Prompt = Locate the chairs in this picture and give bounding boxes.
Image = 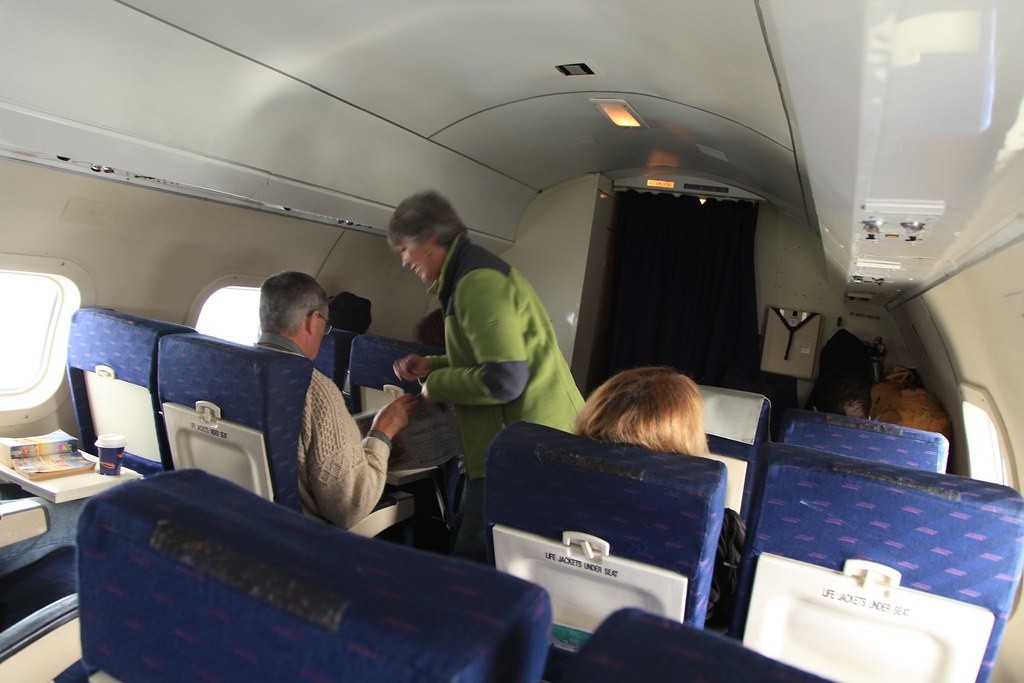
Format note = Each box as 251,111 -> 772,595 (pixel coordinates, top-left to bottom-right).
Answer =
1,305 -> 1024,683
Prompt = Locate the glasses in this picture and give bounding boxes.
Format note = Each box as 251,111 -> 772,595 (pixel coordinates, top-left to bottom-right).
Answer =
308,312 -> 334,335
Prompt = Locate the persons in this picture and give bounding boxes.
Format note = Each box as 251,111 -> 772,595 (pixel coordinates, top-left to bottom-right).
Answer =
253,272 -> 422,543
569,366 -> 746,633
385,188 -> 586,563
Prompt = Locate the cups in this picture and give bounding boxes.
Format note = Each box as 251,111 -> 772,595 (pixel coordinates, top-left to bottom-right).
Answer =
94,434 -> 127,476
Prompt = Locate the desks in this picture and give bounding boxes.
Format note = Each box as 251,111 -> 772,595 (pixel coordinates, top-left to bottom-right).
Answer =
1,450 -> 140,505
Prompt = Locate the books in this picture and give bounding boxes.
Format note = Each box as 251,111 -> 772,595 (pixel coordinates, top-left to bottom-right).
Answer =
0,427 -> 96,481
